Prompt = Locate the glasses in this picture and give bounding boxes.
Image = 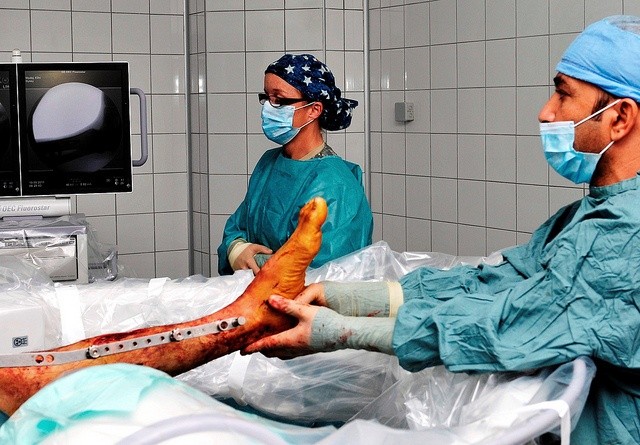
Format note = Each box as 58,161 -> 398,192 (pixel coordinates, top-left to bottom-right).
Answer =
258,92 -> 310,107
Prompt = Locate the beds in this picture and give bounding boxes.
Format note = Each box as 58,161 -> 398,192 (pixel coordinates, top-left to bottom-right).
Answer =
2,244 -> 584,445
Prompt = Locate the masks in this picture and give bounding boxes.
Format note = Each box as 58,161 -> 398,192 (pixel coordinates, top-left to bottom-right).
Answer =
260,99 -> 317,145
538,98 -> 622,184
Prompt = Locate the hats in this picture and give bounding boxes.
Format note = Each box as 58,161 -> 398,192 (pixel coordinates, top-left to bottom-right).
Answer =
555,15 -> 640,100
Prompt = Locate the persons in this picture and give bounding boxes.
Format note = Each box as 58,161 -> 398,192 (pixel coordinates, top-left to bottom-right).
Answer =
217,53 -> 373,276
240,13 -> 640,445
0,195 -> 328,429
